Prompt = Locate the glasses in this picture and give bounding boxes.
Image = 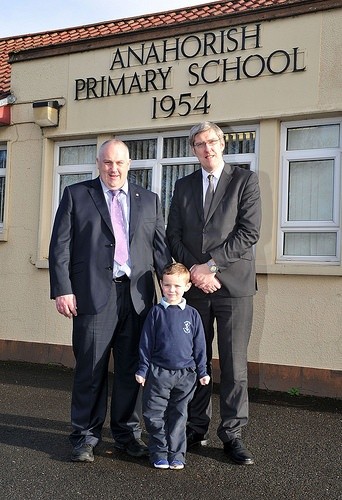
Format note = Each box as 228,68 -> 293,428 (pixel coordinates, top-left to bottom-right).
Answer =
194,139 -> 220,148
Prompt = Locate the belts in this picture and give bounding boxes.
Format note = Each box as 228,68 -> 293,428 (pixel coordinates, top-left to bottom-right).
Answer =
114,274 -> 130,283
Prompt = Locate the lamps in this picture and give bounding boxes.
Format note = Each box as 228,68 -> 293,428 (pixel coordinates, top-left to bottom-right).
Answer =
33,101 -> 61,127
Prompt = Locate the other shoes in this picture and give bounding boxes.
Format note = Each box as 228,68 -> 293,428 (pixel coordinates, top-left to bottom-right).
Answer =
169,461 -> 185,469
154,459 -> 169,469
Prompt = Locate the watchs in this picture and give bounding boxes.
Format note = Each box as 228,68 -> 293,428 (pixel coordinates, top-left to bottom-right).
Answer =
207,261 -> 217,272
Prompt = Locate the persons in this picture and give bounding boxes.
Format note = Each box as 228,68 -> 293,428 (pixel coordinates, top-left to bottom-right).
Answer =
135,264 -> 210,469
48,138 -> 173,462
166,121 -> 261,465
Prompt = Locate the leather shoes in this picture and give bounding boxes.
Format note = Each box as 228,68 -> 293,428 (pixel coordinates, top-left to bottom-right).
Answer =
71,443 -> 95,462
186,427 -> 208,448
224,438 -> 255,465
114,438 -> 150,457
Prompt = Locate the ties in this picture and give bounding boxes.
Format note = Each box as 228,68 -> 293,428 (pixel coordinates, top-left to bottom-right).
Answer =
108,189 -> 130,266
204,175 -> 214,222
